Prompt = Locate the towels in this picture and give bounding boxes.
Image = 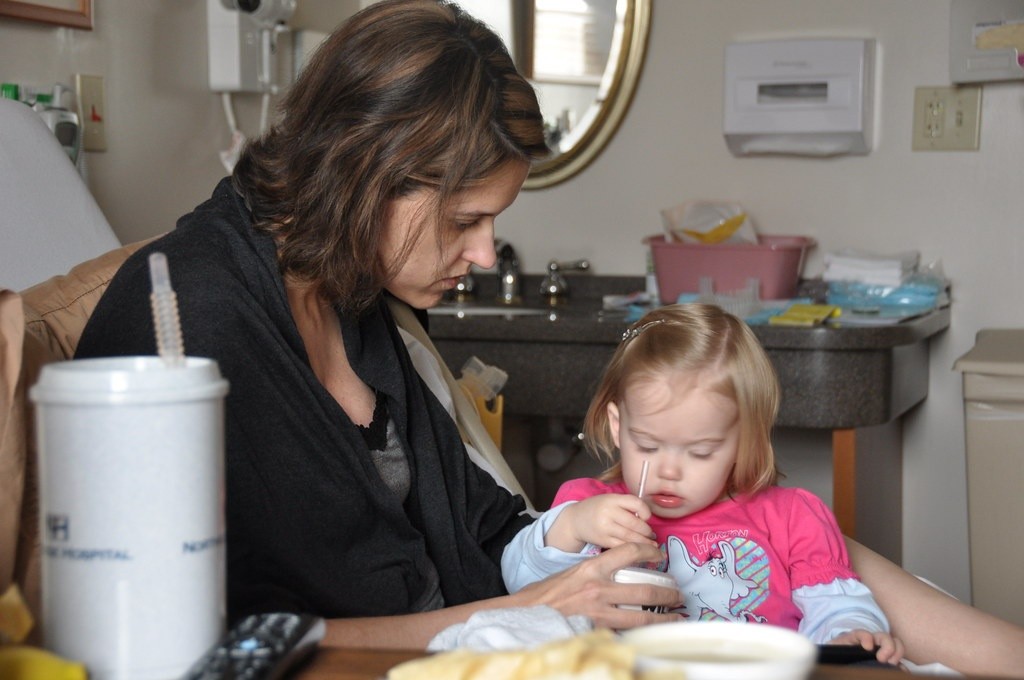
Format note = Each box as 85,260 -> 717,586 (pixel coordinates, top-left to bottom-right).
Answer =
424,601 -> 594,654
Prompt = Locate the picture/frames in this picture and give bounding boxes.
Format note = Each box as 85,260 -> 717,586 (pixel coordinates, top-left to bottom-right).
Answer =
0,0 -> 94,30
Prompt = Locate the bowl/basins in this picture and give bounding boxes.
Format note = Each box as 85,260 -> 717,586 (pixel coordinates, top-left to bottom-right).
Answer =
618,621 -> 817,680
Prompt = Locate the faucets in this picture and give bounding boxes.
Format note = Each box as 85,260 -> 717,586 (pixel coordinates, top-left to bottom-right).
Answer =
490,235 -> 526,308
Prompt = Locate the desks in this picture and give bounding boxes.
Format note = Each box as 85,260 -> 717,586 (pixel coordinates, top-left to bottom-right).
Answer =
287,648 -> 915,680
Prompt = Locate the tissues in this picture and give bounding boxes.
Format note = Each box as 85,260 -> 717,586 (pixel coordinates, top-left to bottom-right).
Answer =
721,34 -> 879,160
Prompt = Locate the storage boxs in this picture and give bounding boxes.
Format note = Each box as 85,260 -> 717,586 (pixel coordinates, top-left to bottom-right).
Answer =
640,232 -> 815,306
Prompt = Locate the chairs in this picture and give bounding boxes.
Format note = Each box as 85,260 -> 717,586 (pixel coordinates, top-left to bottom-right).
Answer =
0,98 -> 541,539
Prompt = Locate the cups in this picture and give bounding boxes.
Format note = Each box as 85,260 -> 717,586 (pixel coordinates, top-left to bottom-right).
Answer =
28,355 -> 230,680
612,566 -> 676,636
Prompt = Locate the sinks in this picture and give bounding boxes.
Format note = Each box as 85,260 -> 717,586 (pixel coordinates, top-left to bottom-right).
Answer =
425,303 -> 552,318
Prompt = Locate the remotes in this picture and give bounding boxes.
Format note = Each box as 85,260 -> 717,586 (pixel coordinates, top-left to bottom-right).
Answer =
182,611 -> 326,680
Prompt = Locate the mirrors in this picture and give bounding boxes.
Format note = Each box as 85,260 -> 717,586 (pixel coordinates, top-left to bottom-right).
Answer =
358,0 -> 652,191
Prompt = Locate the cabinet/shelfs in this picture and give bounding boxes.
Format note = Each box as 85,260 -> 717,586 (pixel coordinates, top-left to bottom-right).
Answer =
954,326 -> 1023,627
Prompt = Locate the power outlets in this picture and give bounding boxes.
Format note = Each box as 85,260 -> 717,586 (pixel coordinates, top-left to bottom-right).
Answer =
910,85 -> 982,151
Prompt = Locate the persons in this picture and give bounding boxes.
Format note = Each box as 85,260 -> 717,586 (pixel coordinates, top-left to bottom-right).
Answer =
499,302 -> 905,666
71,0 -> 1024,680
